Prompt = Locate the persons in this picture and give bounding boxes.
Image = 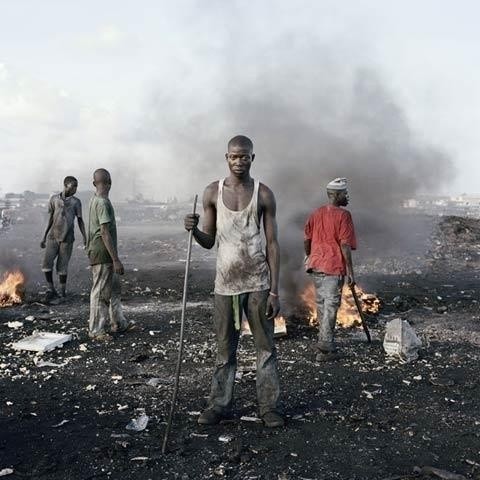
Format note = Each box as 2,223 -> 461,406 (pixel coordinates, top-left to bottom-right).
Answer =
40,168 -> 138,341
184,135 -> 286,427
304,177 -> 357,363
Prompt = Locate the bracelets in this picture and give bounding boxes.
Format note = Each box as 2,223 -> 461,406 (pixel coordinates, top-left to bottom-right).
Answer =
269,291 -> 279,297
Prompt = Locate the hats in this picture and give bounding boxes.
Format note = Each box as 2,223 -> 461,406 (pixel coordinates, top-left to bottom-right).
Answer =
326,177 -> 348,190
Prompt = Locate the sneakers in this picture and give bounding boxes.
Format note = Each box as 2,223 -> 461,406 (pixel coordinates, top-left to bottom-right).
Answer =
197,407 -> 218,426
53,291 -> 65,299
40,290 -> 55,302
316,350 -> 343,363
264,414 -> 284,428
